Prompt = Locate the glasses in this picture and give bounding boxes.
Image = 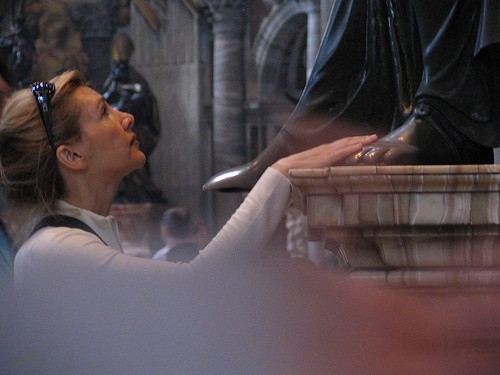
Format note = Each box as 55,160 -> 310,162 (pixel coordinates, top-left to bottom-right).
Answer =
30,82 -> 57,159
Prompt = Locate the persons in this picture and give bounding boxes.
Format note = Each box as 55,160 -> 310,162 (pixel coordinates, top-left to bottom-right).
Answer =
150,206 -> 202,264
190,218 -> 208,251
200,0 -> 500,193
0,69 -> 376,314
